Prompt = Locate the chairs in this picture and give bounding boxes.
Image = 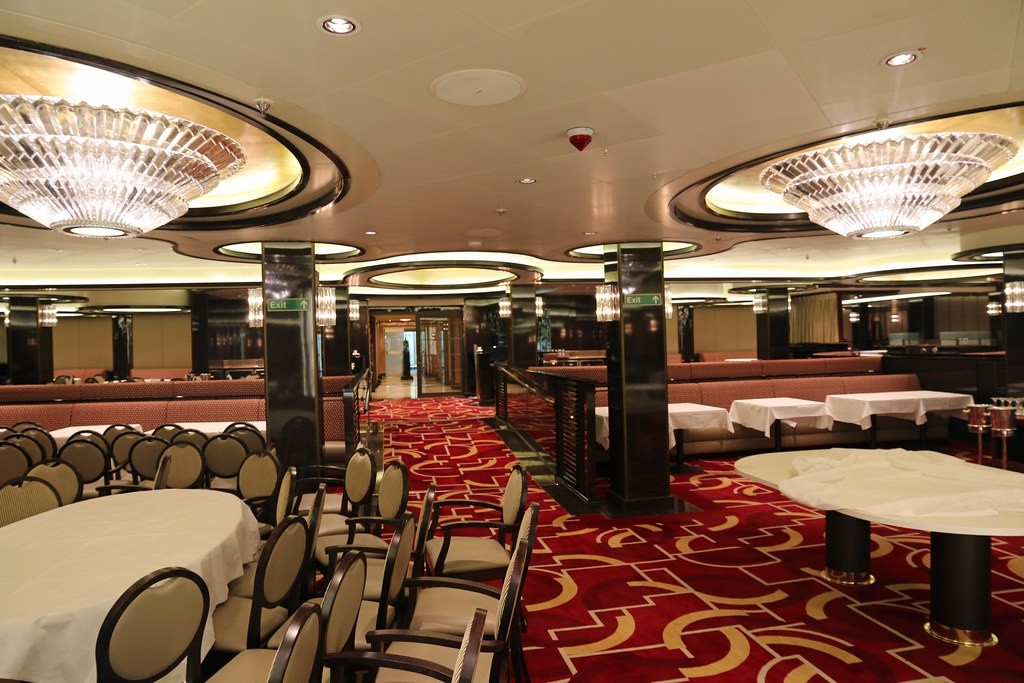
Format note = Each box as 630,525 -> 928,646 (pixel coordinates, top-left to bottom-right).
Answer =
0,422 -> 539,683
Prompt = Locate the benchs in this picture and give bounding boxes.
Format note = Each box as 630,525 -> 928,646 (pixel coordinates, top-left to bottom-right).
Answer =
526,350 -> 919,457
0,368 -> 370,468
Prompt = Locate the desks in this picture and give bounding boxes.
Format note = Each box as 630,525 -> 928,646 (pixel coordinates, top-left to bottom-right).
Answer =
734,449 -> 1024,648
595,402 -> 734,478
0,489 -> 261,683
48,424 -> 144,454
144,422 -> 266,490
825,390 -> 974,453
729,397 -> 834,455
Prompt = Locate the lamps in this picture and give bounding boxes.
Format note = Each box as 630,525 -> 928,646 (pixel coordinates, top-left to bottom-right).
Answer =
42,305 -> 58,328
0,95 -> 246,239
247,288 -> 263,329
499,293 -> 511,319
595,282 -> 620,323
316,285 -> 336,326
349,300 -> 359,322
753,293 -> 768,314
760,134 -> 1019,240
